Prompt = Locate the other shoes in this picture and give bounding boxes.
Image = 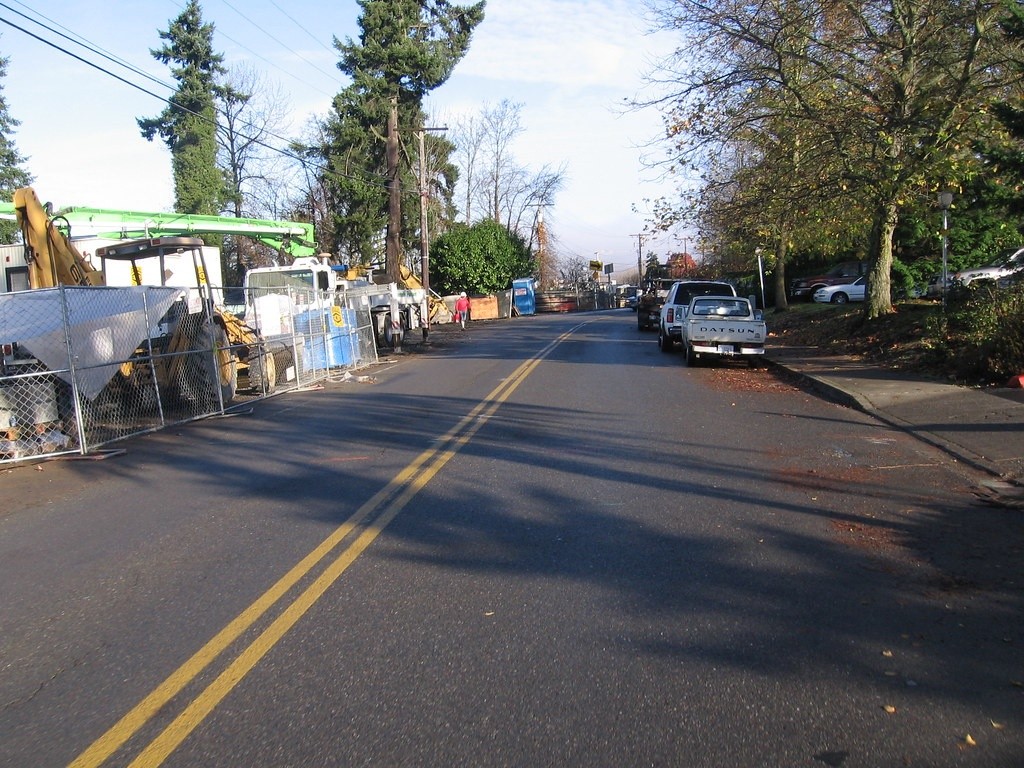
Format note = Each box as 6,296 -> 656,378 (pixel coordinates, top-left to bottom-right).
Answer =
461,328 -> 465,331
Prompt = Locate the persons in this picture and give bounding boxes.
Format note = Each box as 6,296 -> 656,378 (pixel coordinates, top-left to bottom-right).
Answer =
465,294 -> 471,326
455,291 -> 471,330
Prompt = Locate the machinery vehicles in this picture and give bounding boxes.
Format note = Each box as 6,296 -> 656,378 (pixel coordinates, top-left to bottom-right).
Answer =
9,187 -> 278,424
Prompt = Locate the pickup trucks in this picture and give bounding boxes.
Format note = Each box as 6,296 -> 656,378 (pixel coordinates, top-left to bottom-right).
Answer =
681,295 -> 768,369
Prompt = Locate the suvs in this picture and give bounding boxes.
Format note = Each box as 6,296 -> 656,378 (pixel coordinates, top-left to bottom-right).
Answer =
788,261 -> 877,300
657,279 -> 740,352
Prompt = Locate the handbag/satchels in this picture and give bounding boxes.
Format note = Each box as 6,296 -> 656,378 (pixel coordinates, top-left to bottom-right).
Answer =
454,313 -> 459,320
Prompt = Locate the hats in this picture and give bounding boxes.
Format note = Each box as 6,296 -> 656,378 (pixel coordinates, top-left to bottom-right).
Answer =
461,292 -> 467,297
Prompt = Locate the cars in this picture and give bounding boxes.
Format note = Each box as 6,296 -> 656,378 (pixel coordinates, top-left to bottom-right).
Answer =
623,277 -> 684,332
936,247 -> 1024,298
813,271 -> 928,305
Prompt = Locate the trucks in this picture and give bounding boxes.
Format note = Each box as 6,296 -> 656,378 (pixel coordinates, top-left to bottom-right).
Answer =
244,252 -> 427,350
